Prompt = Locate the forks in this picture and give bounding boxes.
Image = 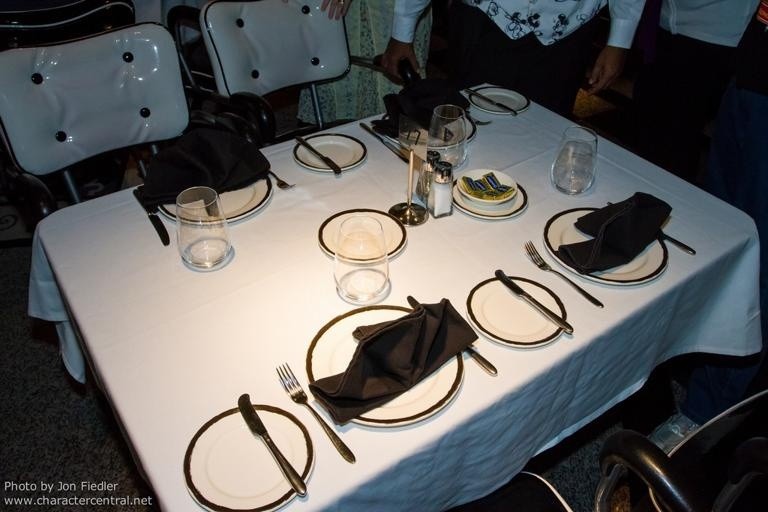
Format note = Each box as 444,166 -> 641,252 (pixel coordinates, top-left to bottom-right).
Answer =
523,241 -> 607,313
269,168 -> 292,194
275,363 -> 359,466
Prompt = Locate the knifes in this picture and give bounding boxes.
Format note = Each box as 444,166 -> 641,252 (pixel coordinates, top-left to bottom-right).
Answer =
493,268 -> 577,335
233,392 -> 307,497
465,86 -> 519,118
125,187 -> 169,251
357,120 -> 410,167
292,134 -> 342,178
407,294 -> 497,377
609,198 -> 697,256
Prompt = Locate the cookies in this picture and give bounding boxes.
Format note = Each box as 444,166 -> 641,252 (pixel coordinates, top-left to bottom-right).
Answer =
462,172 -> 515,200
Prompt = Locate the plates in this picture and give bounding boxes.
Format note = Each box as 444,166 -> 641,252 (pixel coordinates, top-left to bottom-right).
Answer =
184,403 -> 314,509
454,165 -> 519,205
403,112 -> 477,145
470,86 -> 531,114
292,133 -> 367,171
468,277 -> 568,349
161,172 -> 272,224
451,186 -> 530,222
543,207 -> 670,286
307,304 -> 462,428
316,207 -> 408,263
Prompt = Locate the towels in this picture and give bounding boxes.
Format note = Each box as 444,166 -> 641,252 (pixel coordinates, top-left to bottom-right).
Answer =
372,78 -> 472,139
308,295 -> 483,426
558,192 -> 675,272
135,131 -> 271,208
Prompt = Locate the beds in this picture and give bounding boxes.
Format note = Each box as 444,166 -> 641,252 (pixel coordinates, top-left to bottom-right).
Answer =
27,79 -> 761,510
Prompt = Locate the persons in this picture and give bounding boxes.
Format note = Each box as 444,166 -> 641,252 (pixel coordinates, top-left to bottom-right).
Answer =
628,0 -> 763,143
383,0 -> 648,121
316,0 -> 353,22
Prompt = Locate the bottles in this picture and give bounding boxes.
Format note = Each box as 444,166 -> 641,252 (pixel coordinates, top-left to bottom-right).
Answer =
417,150 -> 441,206
426,160 -> 453,218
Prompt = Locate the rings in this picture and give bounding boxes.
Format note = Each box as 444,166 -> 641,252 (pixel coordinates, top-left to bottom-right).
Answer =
338,0 -> 345,5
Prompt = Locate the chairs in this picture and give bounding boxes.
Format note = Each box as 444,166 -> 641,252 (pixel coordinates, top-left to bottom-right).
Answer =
452,465 -> 573,510
596,387 -> 768,512
0,22 -> 190,206
201,1 -> 423,141
0,1 -> 137,48
163,1 -> 218,114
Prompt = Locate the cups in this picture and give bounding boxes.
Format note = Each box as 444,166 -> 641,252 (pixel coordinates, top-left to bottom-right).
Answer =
429,106 -> 469,170
173,187 -> 230,268
551,124 -> 602,194
333,217 -> 391,300
